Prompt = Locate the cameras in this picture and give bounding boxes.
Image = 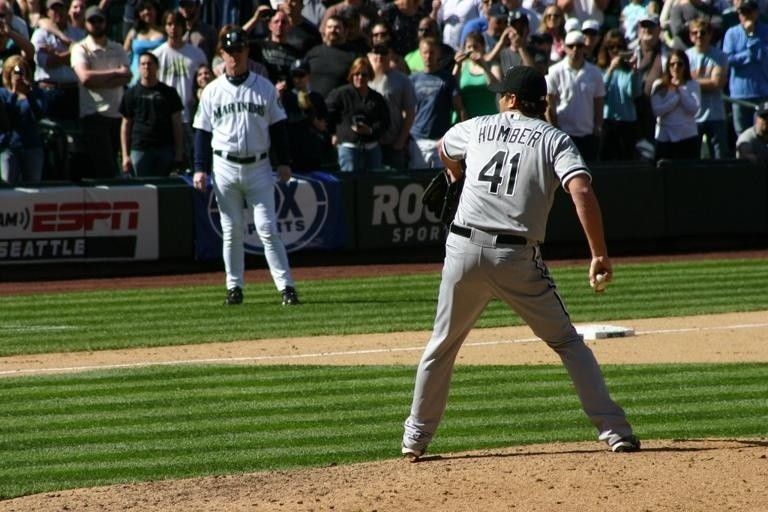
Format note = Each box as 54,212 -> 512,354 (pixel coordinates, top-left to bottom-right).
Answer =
14,65 -> 24,75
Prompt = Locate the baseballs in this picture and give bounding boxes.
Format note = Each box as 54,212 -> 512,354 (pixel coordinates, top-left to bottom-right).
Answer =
591,275 -> 607,291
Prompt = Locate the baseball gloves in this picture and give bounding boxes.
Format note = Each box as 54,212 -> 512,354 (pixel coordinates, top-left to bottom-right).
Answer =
422,171 -> 465,228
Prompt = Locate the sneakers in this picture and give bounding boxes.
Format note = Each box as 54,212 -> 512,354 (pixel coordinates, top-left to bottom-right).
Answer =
611,435 -> 641,452
281,286 -> 298,306
226,286 -> 243,304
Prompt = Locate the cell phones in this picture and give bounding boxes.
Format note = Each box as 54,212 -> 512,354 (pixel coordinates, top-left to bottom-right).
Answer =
260,9 -> 277,16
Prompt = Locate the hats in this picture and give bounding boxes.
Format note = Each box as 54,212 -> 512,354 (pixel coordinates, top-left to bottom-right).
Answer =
46,0 -> 63,9
86,7 -> 106,20
565,17 -> 599,47
757,101 -> 768,115
638,12 -> 659,24
738,1 -> 760,10
222,28 -> 246,53
489,67 -> 547,99
487,4 -> 528,25
289,58 -> 310,75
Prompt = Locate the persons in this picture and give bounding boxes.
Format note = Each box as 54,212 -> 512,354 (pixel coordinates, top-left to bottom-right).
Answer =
0,0 -> 767,191
399,62 -> 642,465
190,29 -> 302,308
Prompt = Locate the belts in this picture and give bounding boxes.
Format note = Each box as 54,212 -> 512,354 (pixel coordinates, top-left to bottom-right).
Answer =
450,222 -> 528,247
214,151 -> 267,165
